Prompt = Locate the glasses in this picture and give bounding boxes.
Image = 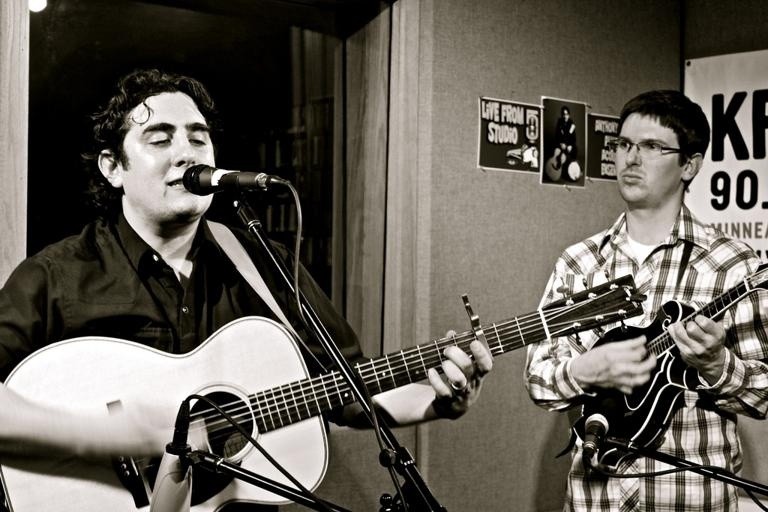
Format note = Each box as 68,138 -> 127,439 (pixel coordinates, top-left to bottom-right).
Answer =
607,135 -> 691,158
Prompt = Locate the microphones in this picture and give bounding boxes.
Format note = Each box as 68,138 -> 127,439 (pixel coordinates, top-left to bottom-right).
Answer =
183,164 -> 269,196
583,414 -> 610,456
149,428 -> 191,512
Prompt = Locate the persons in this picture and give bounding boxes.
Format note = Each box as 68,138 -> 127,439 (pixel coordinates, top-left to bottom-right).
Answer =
552,105 -> 581,178
519,85 -> 768,511
0,65 -> 495,511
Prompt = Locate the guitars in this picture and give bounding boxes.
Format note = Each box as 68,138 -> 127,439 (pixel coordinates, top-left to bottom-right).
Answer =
569,263 -> 768,471
544,124 -> 576,181
1,275 -> 647,510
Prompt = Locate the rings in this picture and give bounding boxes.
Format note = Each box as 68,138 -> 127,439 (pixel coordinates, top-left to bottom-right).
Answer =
452,380 -> 470,394
